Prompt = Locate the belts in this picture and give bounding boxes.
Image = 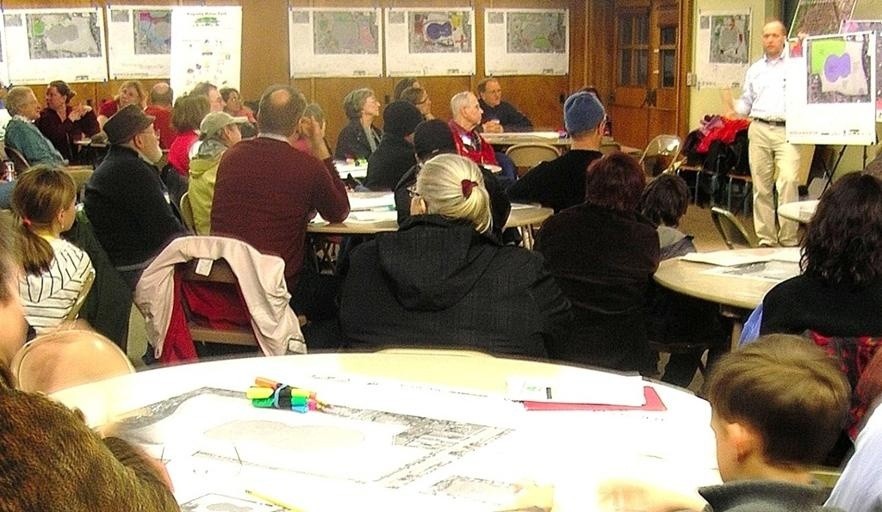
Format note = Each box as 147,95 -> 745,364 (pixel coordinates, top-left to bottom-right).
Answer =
754,116 -> 786,128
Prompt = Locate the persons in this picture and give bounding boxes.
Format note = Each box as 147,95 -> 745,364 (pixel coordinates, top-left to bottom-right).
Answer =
0,76 -> 351,394
734,171 -> 882,350
722,20 -> 810,249
333,78 -> 714,391
694,333 -> 852,512
0,212 -> 183,512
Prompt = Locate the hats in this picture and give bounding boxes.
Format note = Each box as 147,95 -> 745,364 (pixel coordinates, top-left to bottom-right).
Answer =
102,102 -> 156,147
198,110 -> 248,142
561,90 -> 607,135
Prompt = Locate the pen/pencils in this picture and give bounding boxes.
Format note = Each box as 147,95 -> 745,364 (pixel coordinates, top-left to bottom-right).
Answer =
315,401 -> 335,412
246,377 -> 315,414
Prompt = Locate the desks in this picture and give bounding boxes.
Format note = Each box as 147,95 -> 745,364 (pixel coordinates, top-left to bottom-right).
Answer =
1,121 -> 578,257
42,352 -> 722,510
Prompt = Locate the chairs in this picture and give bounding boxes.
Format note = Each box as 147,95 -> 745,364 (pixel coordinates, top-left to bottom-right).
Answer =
777,200 -> 819,246
650,247 -> 806,350
8,329 -> 137,398
709,206 -> 752,247
64,203 -> 158,367
165,233 -> 311,357
601,118 -> 831,216
